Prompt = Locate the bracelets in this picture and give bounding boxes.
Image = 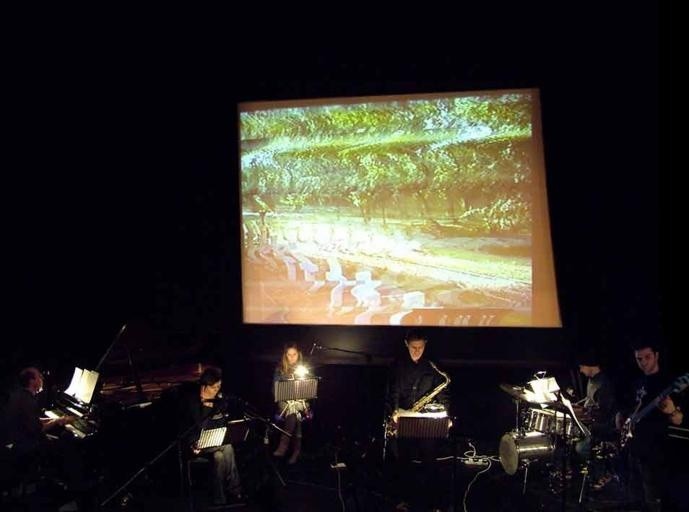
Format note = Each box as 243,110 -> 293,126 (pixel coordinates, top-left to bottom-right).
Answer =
58,417 -> 64,427
670,406 -> 680,419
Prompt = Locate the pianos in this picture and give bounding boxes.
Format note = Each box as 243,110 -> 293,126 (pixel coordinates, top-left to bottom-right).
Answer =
38,388 -> 97,440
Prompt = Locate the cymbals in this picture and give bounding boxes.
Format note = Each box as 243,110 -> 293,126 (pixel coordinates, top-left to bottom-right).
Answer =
500,382 -> 538,403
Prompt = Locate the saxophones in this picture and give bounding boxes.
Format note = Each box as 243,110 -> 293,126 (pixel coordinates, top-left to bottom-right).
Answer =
386,362 -> 451,437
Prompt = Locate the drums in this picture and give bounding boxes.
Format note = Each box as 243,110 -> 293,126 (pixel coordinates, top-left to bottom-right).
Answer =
499,429 -> 552,479
522,408 -> 573,439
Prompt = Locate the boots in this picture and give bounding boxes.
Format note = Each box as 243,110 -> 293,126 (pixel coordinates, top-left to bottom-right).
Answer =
274,434 -> 290,457
288,438 -> 301,464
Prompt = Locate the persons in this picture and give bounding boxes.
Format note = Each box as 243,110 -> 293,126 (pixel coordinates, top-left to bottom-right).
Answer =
270,341 -> 325,466
615,342 -> 683,507
7,366 -> 79,493
570,356 -> 617,470
175,367 -> 250,503
383,328 -> 453,444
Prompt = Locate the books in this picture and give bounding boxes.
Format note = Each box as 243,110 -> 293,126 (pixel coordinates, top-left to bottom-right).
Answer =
529,378 -> 562,408
560,393 -> 591,438
63,367 -> 100,404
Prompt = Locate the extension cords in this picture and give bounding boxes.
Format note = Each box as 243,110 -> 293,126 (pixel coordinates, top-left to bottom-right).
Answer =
330,463 -> 346,468
464,456 -> 488,466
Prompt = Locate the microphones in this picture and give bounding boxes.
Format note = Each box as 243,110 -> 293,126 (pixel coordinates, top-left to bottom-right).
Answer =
310,342 -> 317,355
205,398 -> 229,405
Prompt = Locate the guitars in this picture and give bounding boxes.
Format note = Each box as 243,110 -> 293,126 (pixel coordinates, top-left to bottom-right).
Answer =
618,373 -> 687,450
